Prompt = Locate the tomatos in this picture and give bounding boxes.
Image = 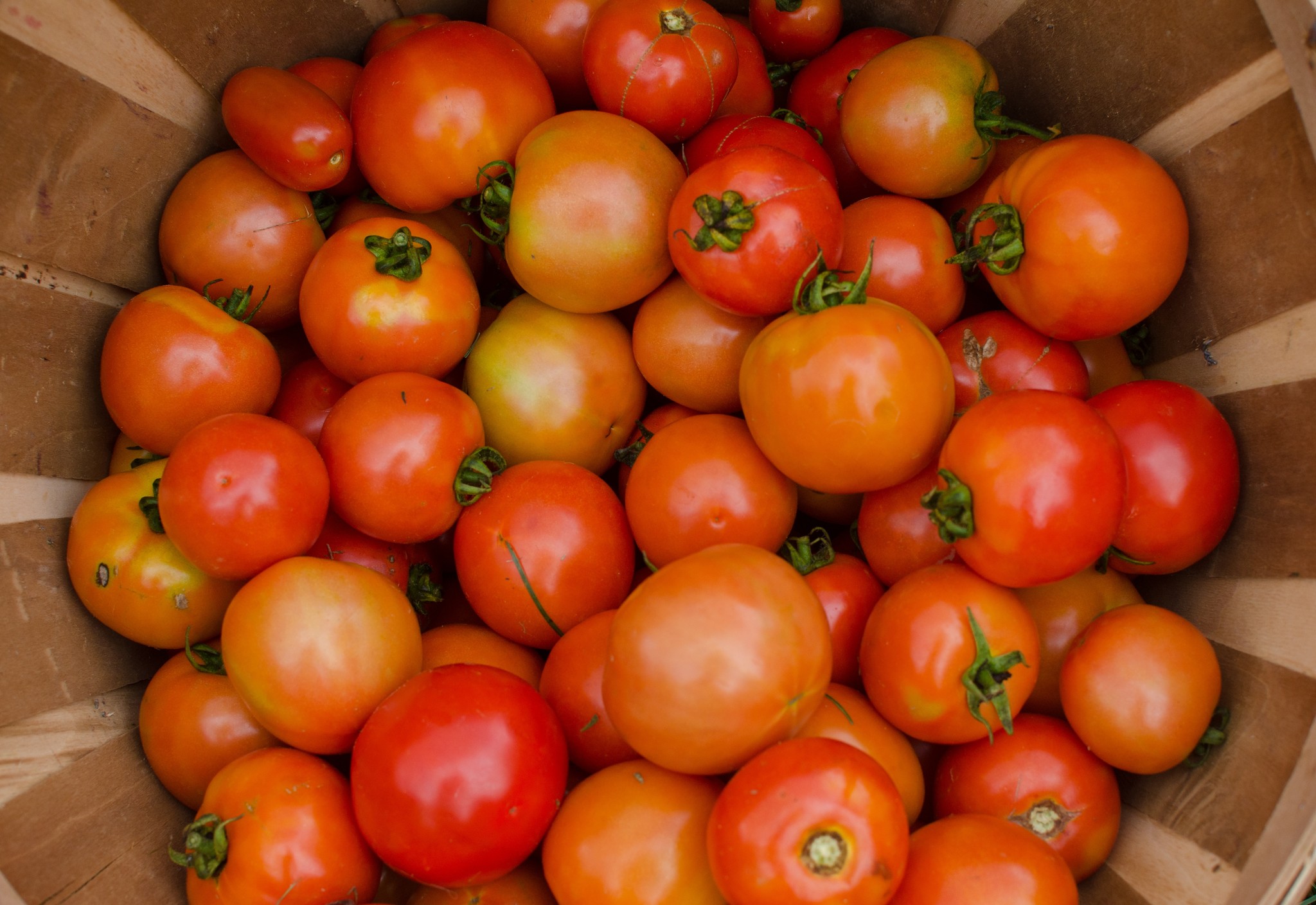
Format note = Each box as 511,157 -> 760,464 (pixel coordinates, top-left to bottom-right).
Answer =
63,4 -> 1252,904
